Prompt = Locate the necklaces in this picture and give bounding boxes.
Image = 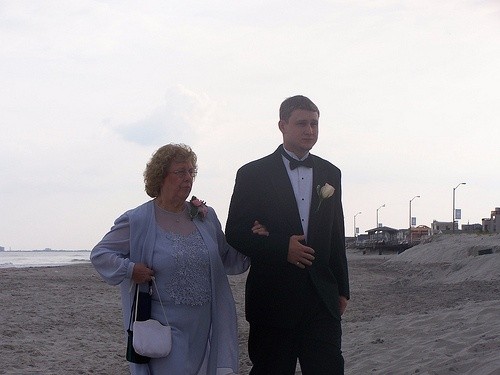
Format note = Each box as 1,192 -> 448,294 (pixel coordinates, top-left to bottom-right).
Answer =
155,201 -> 190,223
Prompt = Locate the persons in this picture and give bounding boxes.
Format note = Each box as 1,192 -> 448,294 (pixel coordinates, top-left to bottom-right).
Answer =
224,95 -> 350,375
89,145 -> 269,375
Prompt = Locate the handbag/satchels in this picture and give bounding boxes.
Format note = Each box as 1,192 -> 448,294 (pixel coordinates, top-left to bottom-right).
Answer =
126,276 -> 172,365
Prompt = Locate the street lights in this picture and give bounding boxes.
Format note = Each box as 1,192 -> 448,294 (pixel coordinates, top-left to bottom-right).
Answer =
409,194 -> 420,245
452,182 -> 467,233
376,203 -> 386,242
353,211 -> 362,243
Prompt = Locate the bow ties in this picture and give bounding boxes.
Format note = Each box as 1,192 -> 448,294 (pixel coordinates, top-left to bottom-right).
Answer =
280,144 -> 314,170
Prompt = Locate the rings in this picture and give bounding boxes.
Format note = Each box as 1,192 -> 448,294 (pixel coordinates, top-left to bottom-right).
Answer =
295,261 -> 299,266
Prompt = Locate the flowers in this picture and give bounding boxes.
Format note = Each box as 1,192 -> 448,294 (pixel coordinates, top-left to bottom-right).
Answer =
189,196 -> 209,223
316,183 -> 336,210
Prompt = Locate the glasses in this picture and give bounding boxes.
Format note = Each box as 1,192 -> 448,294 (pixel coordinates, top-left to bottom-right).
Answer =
169,169 -> 197,177
